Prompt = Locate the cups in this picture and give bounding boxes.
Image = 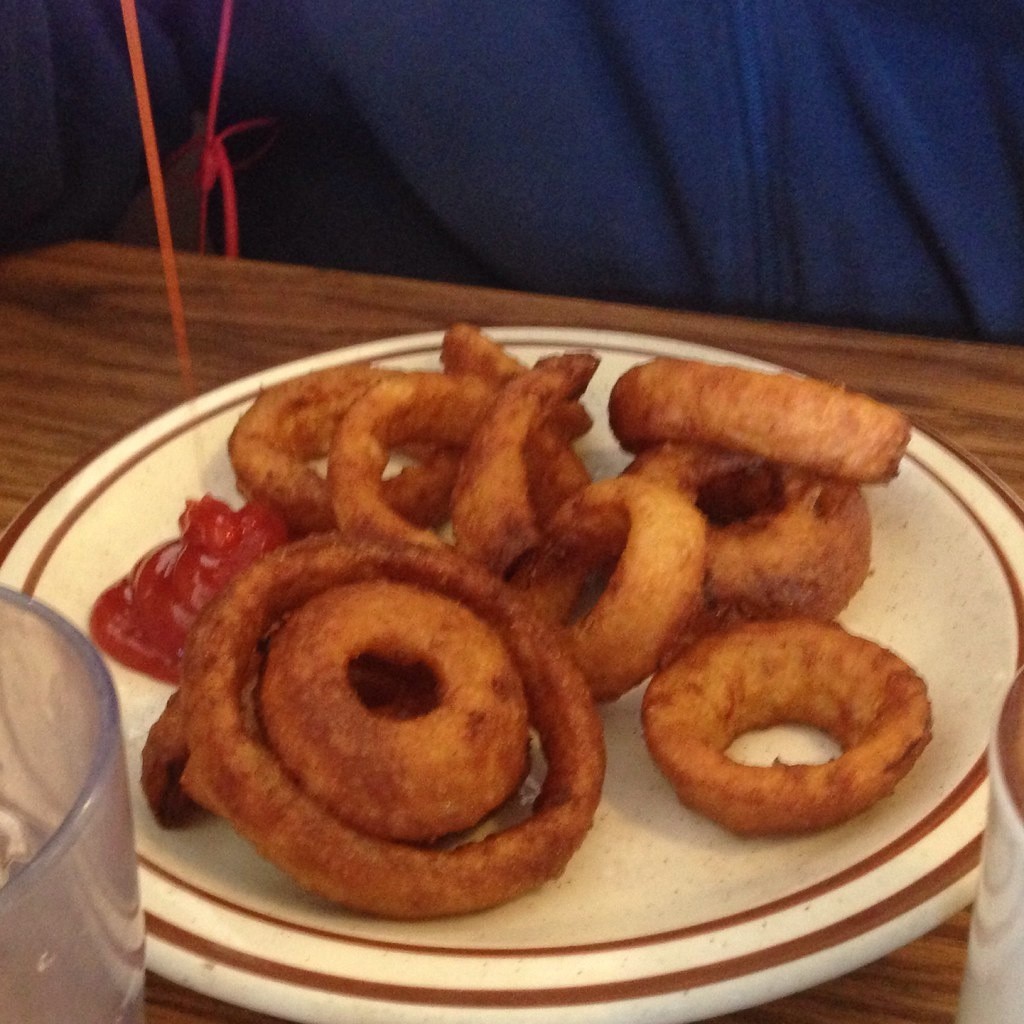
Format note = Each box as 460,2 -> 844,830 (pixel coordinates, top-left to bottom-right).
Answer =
0,585 -> 147,1024
958,667 -> 1024,1024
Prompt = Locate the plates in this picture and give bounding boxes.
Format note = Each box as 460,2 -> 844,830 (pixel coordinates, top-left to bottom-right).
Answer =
0,327 -> 1024,1024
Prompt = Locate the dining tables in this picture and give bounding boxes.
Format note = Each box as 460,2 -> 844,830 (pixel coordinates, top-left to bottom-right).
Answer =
1,240 -> 1023,1023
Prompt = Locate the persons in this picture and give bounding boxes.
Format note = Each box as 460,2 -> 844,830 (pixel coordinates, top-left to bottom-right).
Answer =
0,0 -> 1024,347
